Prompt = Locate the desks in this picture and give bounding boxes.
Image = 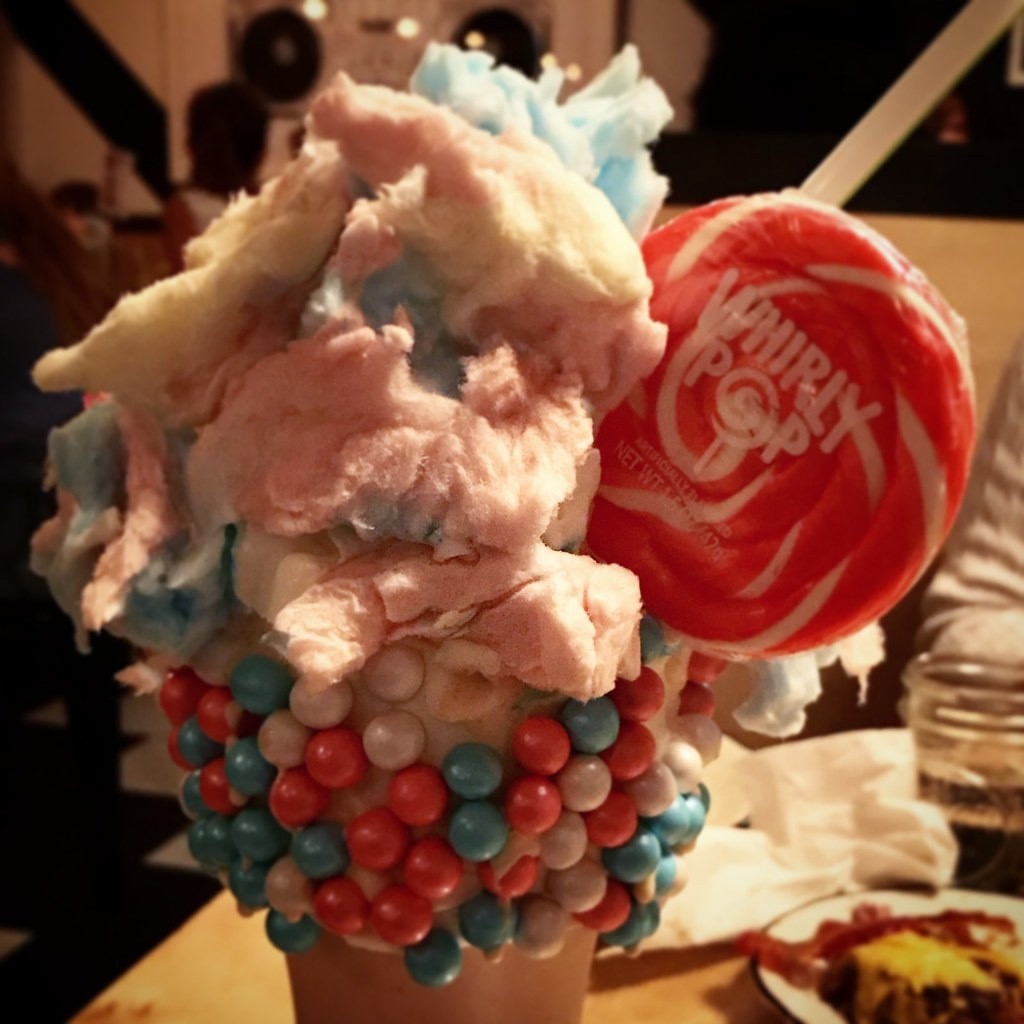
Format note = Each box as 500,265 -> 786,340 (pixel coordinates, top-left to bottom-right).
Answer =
60,214 -> 1024,1024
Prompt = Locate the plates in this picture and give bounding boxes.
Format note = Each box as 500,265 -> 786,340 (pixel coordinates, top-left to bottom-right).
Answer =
752,889 -> 1024,1024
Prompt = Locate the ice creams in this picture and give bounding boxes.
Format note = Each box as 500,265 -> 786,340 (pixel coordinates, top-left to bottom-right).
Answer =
32,42 -> 891,976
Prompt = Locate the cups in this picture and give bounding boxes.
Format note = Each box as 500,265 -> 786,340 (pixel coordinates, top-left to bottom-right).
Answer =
896,653 -> 1024,899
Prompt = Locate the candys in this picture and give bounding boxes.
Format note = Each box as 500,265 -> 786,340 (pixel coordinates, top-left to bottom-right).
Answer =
579,193 -> 976,657
